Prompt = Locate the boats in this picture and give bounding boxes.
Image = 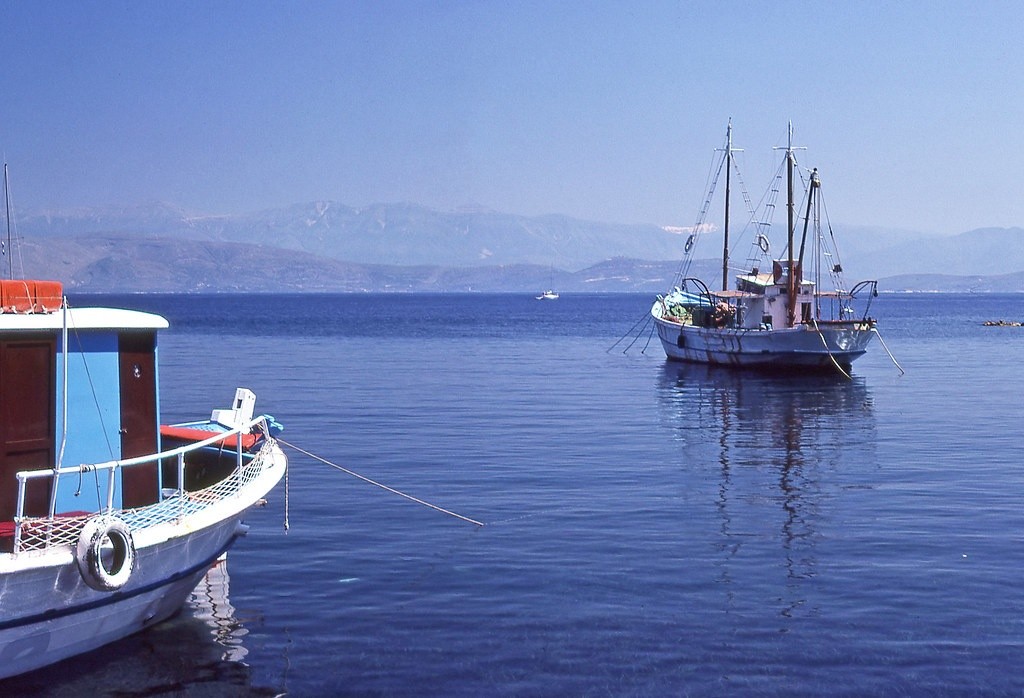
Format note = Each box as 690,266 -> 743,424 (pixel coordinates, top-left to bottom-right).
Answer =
0,278 -> 290,680
534,289 -> 560,300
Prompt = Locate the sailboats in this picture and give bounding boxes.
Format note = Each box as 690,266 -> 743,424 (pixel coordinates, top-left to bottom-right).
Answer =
649,114 -> 878,368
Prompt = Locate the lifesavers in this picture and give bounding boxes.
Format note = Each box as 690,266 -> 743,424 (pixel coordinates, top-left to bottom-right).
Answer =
76,516 -> 136,592
758,235 -> 770,253
685,235 -> 694,252
677,336 -> 685,349
